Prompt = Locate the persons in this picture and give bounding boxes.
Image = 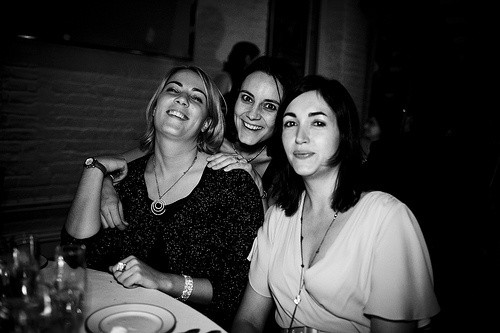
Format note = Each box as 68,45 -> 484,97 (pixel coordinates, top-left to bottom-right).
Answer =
232,74 -> 441,332
99,56 -> 301,231
223,41 -> 259,76
61,64 -> 264,333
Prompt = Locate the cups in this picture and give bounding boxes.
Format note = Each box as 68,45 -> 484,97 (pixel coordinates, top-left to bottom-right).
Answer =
0,234 -> 86,333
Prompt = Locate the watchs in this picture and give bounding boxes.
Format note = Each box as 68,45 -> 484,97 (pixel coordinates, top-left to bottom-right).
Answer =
84,157 -> 107,175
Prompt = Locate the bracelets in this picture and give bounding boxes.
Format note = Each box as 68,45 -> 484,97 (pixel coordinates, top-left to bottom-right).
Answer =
175,274 -> 193,302
109,174 -> 114,180
261,191 -> 267,199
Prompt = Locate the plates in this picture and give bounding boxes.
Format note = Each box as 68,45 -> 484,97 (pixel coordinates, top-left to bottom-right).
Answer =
84,303 -> 176,333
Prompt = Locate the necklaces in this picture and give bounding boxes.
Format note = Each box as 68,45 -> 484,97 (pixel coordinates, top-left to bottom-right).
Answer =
233,143 -> 265,163
294,191 -> 337,305
151,150 -> 198,215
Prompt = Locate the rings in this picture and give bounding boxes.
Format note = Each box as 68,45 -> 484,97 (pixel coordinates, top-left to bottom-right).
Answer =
233,157 -> 240,163
116,263 -> 126,273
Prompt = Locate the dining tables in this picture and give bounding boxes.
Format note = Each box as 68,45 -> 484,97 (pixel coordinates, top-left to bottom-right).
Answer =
41,260 -> 230,333
